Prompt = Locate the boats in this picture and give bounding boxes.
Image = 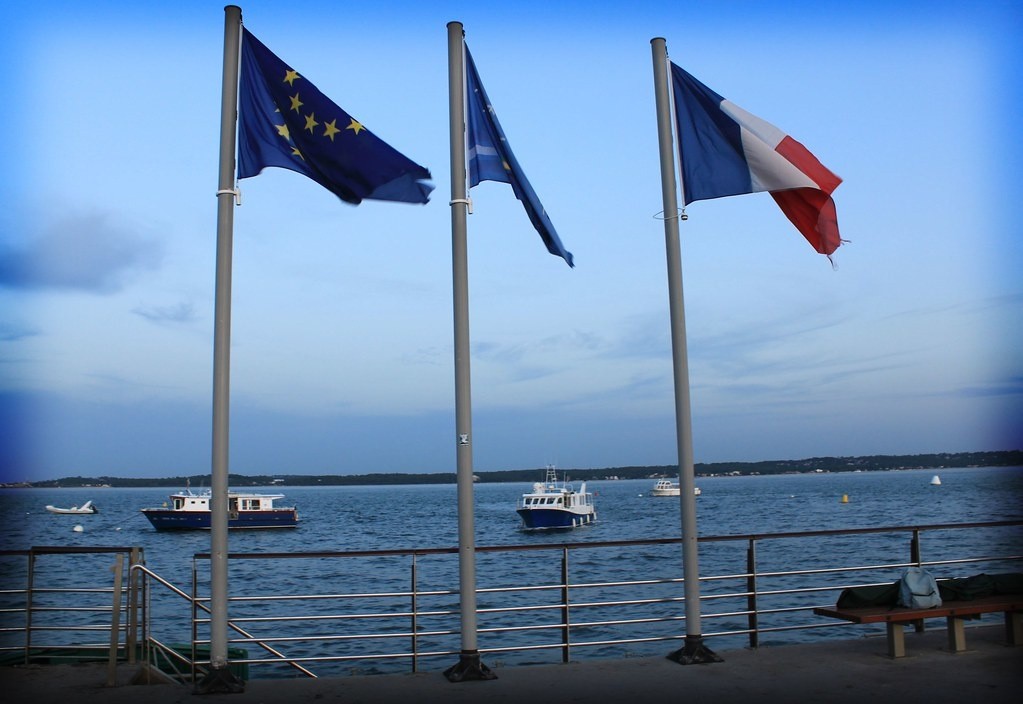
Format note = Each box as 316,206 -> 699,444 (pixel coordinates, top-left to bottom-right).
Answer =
45,501 -> 98,514
516,464 -> 597,529
140,478 -> 298,532
650,477 -> 701,496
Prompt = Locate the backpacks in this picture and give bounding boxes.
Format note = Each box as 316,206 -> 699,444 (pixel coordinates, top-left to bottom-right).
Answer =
901,566 -> 942,610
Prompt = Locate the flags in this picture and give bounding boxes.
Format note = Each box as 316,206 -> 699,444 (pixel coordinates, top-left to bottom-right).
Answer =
242,26 -> 436,206
464,40 -> 576,270
671,60 -> 843,257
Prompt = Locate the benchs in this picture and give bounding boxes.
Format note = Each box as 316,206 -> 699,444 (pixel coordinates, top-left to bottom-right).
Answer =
813,573 -> 1023,658
